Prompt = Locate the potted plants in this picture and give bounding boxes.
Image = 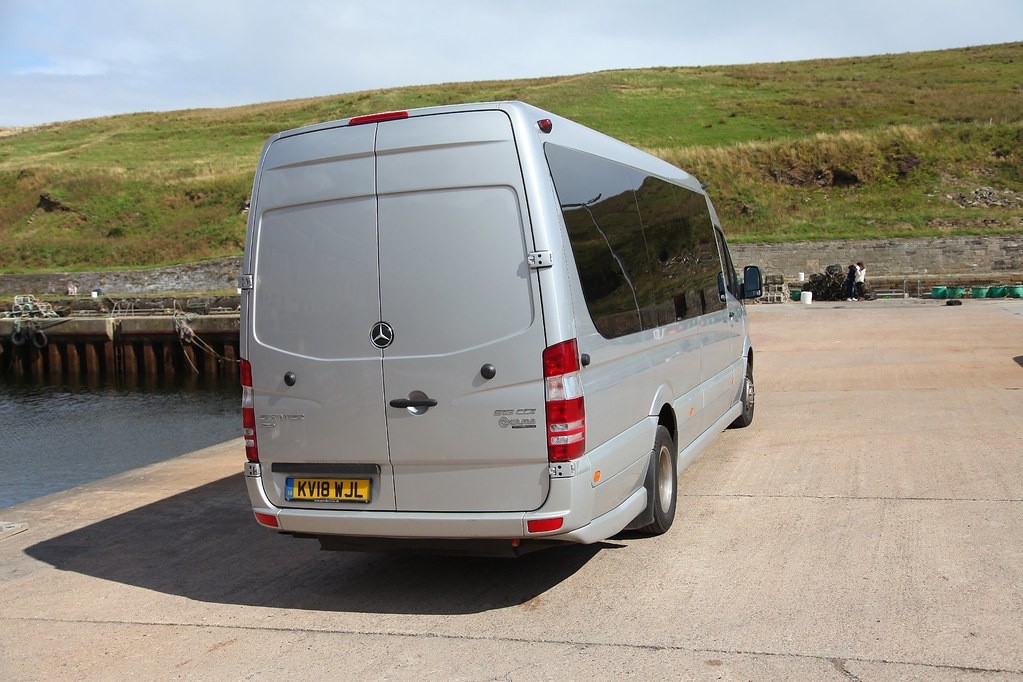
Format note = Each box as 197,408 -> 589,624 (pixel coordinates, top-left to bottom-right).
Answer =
990,284 -> 1005,298
1005,285 -> 1023,298
930,280 -> 946,298
971,283 -> 990,298
947,279 -> 966,299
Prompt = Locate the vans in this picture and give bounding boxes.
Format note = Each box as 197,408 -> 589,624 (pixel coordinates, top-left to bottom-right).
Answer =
238,99 -> 763,560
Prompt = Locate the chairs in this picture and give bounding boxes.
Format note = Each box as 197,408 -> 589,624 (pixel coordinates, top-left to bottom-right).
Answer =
767,285 -> 784,303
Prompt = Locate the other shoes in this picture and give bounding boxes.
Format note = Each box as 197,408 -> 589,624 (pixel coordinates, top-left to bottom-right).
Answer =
852,298 -> 857,301
847,298 -> 852,302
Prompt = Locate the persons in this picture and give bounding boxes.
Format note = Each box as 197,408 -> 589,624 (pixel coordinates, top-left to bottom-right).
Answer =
845,262 -> 866,302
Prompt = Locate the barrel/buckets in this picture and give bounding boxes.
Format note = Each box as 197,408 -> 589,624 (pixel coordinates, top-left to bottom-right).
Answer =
801,291 -> 813,305
799,272 -> 805,282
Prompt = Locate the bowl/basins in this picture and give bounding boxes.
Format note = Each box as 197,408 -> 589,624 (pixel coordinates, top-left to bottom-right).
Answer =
929,285 -> 1023,300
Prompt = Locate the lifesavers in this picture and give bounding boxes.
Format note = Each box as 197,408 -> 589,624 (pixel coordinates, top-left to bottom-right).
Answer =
11,327 -> 27,346
32,332 -> 48,348
